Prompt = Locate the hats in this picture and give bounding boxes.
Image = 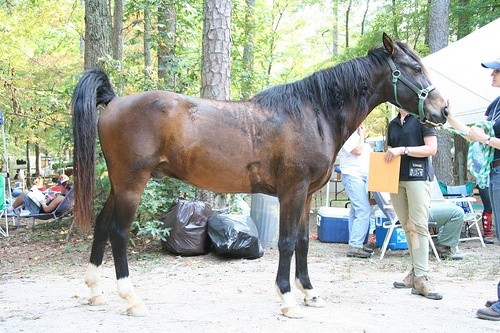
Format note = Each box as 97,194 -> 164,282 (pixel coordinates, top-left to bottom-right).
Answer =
55,174 -> 70,186
481,60 -> 500,69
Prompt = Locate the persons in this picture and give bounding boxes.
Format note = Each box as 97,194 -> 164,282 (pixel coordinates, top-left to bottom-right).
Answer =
33,173 -> 44,187
339,121 -> 374,259
471,127 -> 500,244
7,173 -> 72,219
387,193 -> 465,261
385,108 -> 445,300
446,61 -> 500,322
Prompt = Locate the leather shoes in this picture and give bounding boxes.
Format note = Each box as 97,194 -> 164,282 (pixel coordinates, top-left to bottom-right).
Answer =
347,245 -> 371,258
393,270 -> 414,288
485,300 -> 500,307
363,243 -> 373,253
411,274 -> 443,299
476,306 -> 500,320
482,233 -> 494,244
432,242 -> 462,260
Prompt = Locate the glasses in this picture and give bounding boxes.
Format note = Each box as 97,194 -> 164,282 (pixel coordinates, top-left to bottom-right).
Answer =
493,68 -> 500,73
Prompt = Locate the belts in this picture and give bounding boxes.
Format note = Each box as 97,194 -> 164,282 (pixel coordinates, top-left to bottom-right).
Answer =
490,160 -> 500,166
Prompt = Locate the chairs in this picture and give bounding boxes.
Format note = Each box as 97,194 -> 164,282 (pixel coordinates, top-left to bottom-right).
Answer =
2,185 -> 76,233
373,147 -> 486,263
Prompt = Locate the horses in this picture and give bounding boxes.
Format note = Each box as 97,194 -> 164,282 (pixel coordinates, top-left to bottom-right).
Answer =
69,31 -> 450,320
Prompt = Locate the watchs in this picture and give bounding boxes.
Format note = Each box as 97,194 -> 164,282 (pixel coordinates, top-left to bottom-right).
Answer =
404,146 -> 409,156
486,136 -> 492,146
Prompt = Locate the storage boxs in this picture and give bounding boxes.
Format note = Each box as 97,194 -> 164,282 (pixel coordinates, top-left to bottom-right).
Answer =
373,205 -> 409,249
316,206 -> 370,243
